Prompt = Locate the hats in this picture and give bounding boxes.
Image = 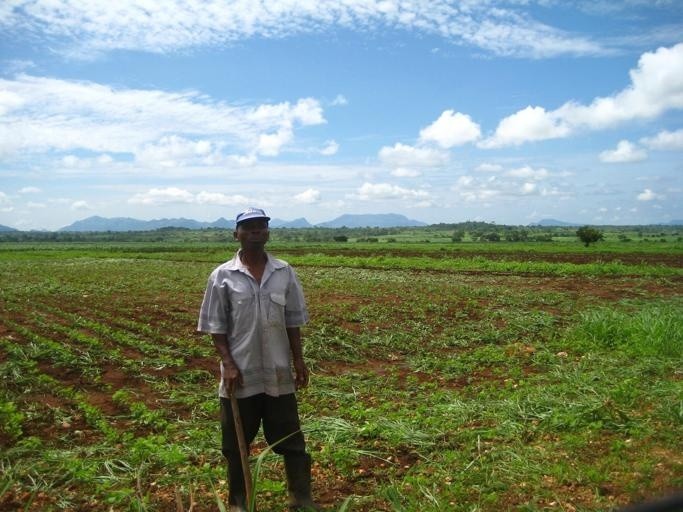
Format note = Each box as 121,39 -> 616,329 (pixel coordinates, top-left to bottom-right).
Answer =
236,207 -> 270,227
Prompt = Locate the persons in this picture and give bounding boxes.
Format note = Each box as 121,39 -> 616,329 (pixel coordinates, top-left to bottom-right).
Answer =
197,208 -> 311,512
